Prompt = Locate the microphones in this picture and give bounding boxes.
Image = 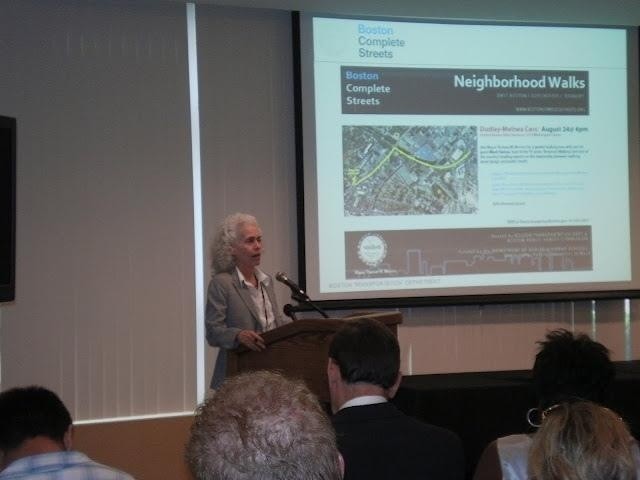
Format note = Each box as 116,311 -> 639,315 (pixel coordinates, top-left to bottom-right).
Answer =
284,304 -> 297,322
276,271 -> 304,296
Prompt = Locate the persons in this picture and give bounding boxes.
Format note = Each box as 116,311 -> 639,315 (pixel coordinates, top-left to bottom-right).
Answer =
526,395 -> 638,480
183,369 -> 346,480
324,317 -> 473,480
473,327 -> 640,480
203,214 -> 285,390
0,385 -> 136,480
